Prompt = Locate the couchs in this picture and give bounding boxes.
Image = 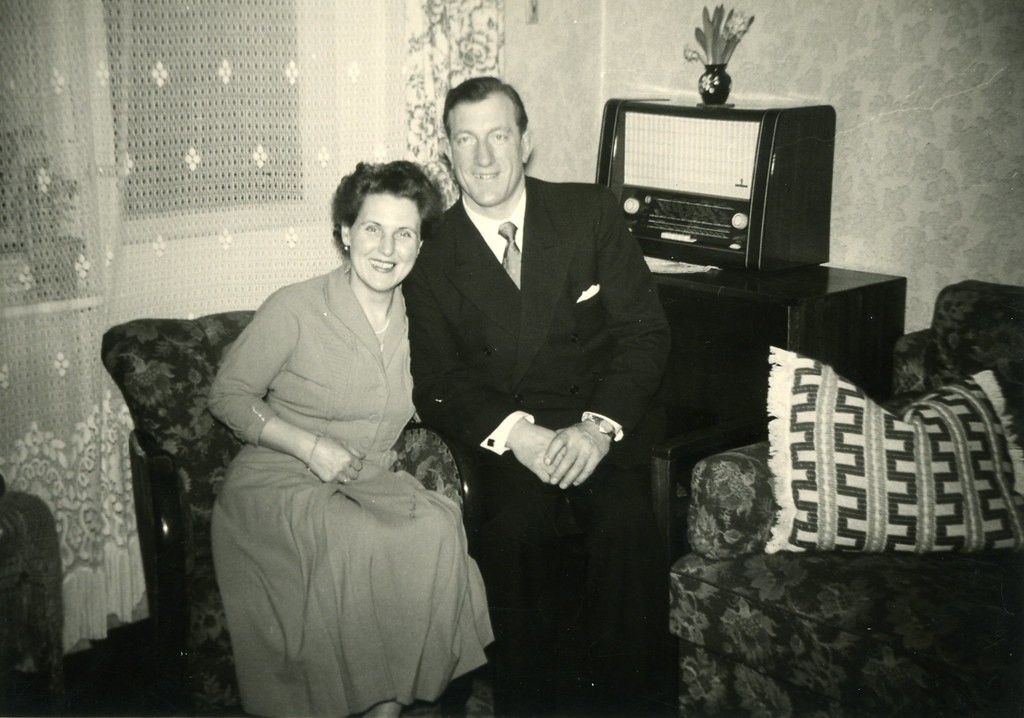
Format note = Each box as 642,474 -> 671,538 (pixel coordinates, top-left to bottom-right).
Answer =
667,281 -> 1023,718
100,310 -> 682,718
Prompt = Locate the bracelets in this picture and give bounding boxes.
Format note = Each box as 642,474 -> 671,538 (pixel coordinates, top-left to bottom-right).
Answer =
306,435 -> 322,471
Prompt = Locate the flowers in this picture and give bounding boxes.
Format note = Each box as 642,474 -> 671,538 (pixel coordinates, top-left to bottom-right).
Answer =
683,4 -> 754,65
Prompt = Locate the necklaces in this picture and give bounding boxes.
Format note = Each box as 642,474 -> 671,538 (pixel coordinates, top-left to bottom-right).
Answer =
374,317 -> 390,334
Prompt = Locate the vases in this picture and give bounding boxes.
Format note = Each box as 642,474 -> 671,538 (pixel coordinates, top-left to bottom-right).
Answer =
26,237 -> 84,301
698,63 -> 732,105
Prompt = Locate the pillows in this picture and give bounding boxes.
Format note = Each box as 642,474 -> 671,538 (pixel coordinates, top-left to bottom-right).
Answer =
765,345 -> 1024,557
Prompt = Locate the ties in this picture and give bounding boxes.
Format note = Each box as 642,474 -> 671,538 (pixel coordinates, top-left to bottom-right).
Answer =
496,221 -> 522,291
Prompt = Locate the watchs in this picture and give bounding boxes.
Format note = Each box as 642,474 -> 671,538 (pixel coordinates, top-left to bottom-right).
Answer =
584,414 -> 616,440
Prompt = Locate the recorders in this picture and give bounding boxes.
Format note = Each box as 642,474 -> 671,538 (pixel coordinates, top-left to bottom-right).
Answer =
595,98 -> 836,270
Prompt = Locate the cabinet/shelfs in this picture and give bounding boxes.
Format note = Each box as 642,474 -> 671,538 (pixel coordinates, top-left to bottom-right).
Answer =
655,266 -> 907,559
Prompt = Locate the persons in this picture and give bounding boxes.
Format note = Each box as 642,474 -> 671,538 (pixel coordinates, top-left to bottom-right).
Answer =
206,161 -> 496,718
402,76 -> 680,718
